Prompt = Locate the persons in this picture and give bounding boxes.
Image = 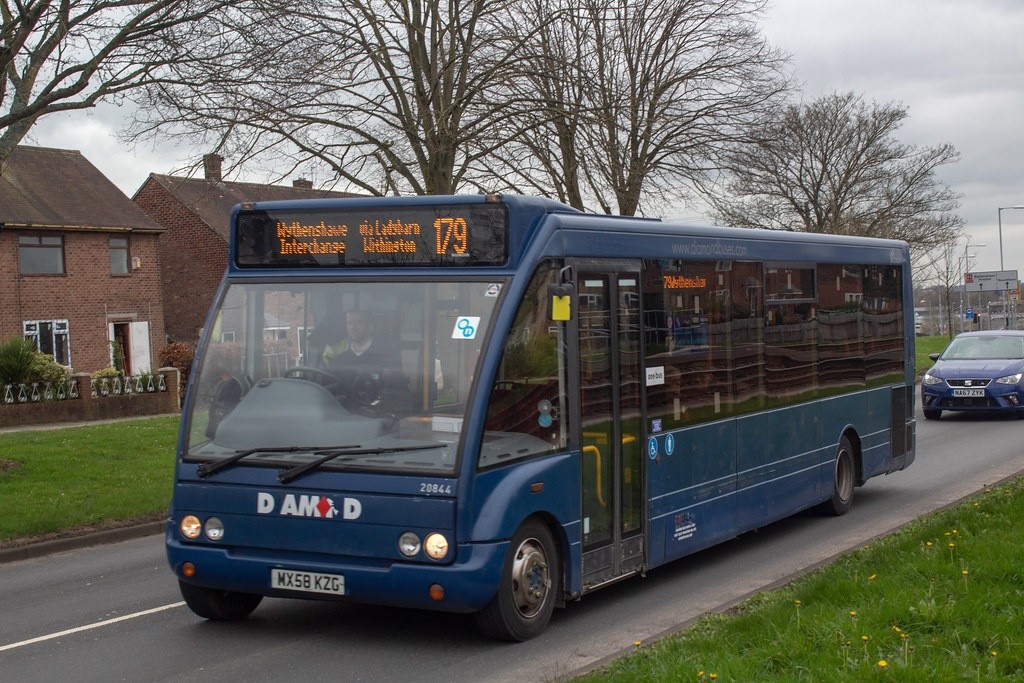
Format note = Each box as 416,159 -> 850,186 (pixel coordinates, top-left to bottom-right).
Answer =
321,300 -> 407,411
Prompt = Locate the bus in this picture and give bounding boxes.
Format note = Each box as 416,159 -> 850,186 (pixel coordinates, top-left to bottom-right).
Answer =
161,190 -> 929,641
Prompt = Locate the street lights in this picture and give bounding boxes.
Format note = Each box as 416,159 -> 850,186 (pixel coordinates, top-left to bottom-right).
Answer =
965,243 -> 987,273
958,255 -> 977,333
998,205 -> 1024,272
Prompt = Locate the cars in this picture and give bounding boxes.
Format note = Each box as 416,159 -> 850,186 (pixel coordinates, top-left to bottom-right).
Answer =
920,329 -> 1024,421
